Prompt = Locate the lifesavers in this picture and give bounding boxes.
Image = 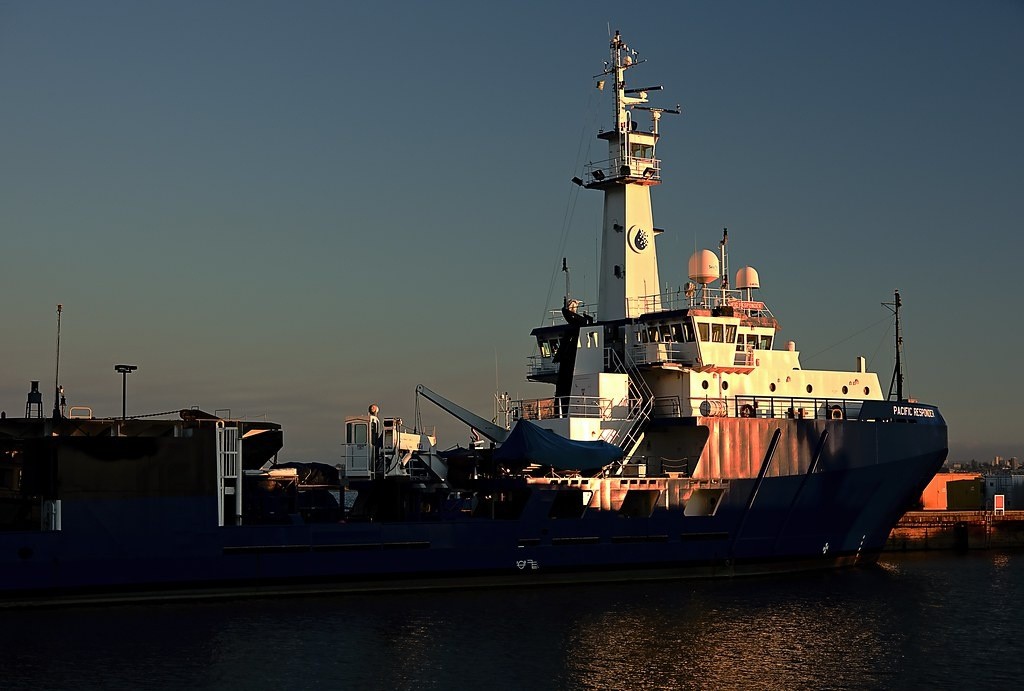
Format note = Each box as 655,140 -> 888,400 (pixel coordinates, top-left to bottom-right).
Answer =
831,408 -> 843,420
740,404 -> 755,418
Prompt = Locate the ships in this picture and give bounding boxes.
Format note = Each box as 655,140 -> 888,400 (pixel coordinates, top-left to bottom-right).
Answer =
0,19 -> 950,604
